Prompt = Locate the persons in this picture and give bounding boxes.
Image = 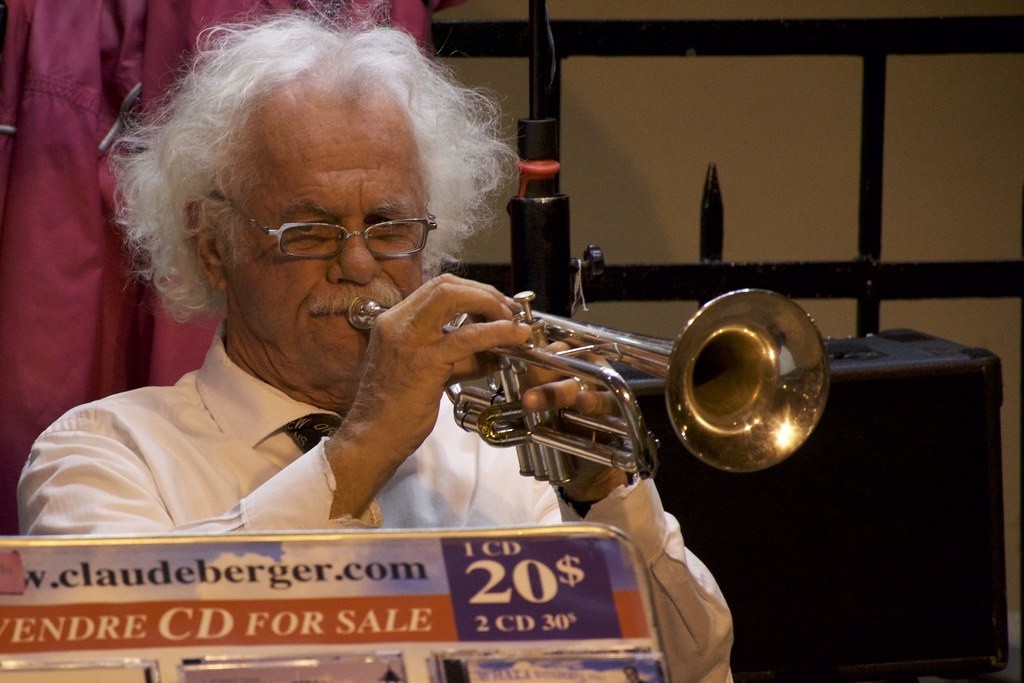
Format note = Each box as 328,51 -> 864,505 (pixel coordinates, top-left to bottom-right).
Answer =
16,2 -> 734,681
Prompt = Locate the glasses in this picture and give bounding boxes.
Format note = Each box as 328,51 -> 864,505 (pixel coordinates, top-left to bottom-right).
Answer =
230,205 -> 438,259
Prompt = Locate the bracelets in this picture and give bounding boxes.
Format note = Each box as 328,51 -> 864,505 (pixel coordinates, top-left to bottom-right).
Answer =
558,468 -> 642,518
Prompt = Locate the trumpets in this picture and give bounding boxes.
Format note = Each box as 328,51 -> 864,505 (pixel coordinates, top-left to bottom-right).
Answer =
348,287 -> 831,503
